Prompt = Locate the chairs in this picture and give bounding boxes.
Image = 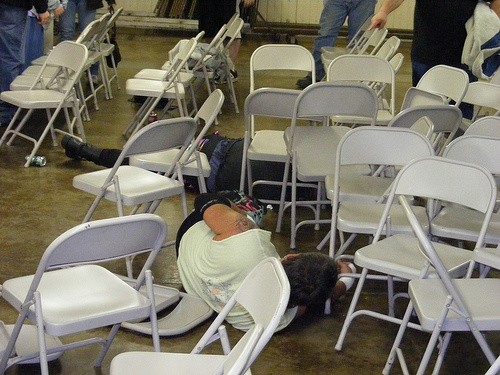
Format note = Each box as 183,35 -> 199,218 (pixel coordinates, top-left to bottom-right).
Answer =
1,213 -> 168,375
128,88 -> 225,215
382,195 -> 500,375
473,241 -> 500,279
275,80 -> 378,249
125,12 -> 244,137
321,12 -> 403,126
109,256 -> 291,375
0,320 -> 64,375
0,7 -> 123,168
240,40 -> 500,249
322,126 -> 435,315
335,156 -> 497,375
72,117 -> 199,279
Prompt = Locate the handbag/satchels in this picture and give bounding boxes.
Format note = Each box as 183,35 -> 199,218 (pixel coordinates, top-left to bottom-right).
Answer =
103,8 -> 121,68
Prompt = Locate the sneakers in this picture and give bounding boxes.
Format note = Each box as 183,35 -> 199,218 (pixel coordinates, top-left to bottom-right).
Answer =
86,74 -> 99,85
214,69 -> 238,84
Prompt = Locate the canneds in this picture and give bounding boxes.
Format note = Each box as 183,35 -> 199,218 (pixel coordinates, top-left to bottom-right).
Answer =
147,111 -> 158,125
24,155 -> 47,167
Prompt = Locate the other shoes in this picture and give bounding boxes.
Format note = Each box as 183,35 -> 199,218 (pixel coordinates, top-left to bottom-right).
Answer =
296,75 -> 320,89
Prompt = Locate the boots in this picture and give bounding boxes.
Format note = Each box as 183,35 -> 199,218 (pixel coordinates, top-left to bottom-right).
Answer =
61,135 -> 103,161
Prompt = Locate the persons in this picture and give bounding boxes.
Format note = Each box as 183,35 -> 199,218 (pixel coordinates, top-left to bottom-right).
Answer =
0,0 -> 69,137
296,0 -> 378,88
58,0 -> 117,85
176,190 -> 356,333
61,134 -> 311,207
198,0 -> 255,83
368,0 -> 500,120
